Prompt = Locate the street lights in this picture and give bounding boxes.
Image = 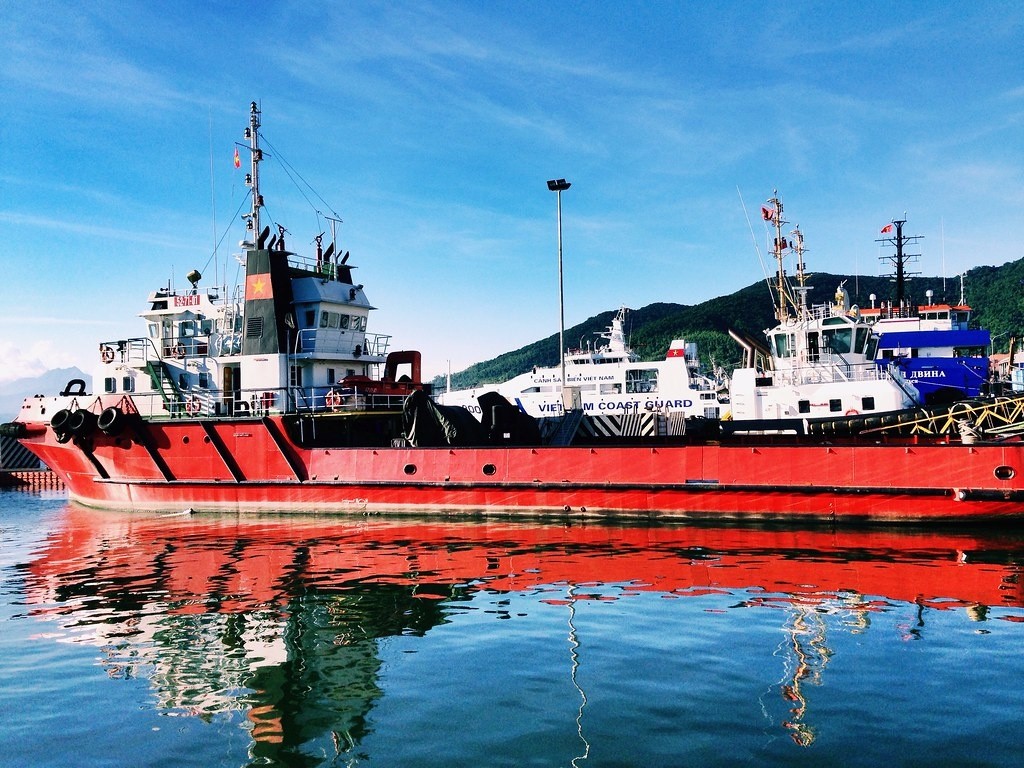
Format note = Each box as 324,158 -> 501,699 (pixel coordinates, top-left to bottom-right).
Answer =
548,177 -> 572,383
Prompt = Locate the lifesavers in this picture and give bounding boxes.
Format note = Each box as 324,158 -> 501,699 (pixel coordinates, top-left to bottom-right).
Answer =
845,409 -> 859,416
49,406 -> 128,436
100,347 -> 114,364
326,391 -> 343,406
186,398 -> 202,415
172,343 -> 186,359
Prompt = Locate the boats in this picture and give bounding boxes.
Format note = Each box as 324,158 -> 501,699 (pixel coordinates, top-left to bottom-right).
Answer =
7,96 -> 1023,523
